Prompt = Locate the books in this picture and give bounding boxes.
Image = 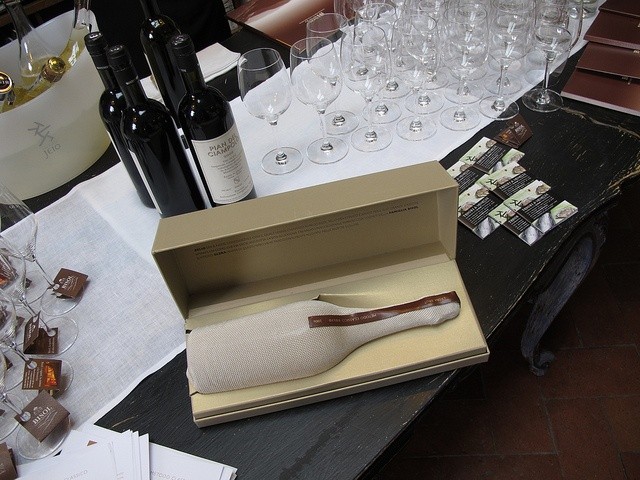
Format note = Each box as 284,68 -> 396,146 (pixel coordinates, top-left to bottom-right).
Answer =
561,0 -> 640,117
224,0 -> 363,54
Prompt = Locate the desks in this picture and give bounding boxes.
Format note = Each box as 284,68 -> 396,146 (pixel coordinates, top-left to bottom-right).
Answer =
0,0 -> 640,480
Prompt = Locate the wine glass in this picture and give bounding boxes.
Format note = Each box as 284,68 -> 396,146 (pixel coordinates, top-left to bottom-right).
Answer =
1,289 -> 73,403
0,351 -> 26,392
0,394 -> 24,442
0,183 -> 84,316
7,270 -> 49,306
0,351 -> 71,460
237,47 -> 302,175
290,37 -> 348,164
0,236 -> 79,357
307,0 -> 600,152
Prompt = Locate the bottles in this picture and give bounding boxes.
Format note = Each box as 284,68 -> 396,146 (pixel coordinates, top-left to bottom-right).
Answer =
2,0 -> 57,86
1,57 -> 67,112
169,34 -> 256,209
0,72 -> 14,114
84,31 -> 155,208
139,0 -> 206,129
106,45 -> 207,218
59,0 -> 91,70
186,290 -> 460,394
